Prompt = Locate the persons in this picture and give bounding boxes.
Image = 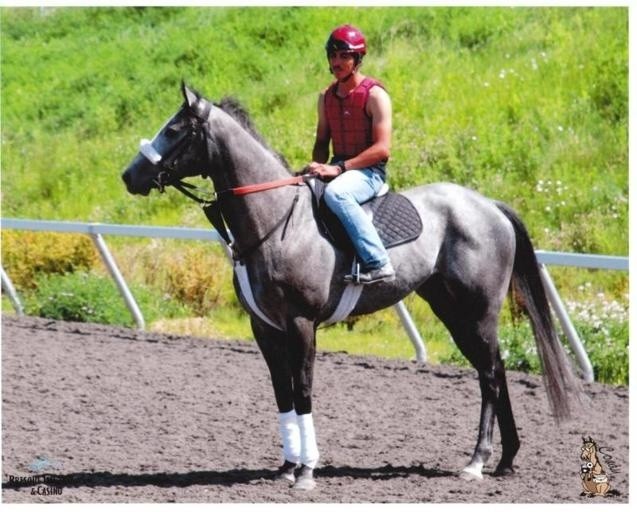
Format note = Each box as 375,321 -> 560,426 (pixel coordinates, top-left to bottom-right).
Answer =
305,25 -> 395,285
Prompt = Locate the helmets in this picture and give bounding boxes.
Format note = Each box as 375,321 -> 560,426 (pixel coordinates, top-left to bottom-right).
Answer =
324,23 -> 368,58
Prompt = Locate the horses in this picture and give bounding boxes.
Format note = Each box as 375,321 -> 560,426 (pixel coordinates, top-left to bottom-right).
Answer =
121,78 -> 584,494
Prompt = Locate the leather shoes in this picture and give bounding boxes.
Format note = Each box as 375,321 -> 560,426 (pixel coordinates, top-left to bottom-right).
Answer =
342,263 -> 397,288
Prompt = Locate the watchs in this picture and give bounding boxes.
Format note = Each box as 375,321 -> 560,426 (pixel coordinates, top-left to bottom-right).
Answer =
338,160 -> 345,173
